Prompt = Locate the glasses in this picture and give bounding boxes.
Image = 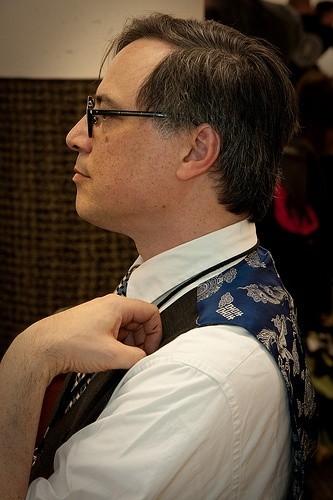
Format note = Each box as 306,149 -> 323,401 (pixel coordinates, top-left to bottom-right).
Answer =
86,96 -> 199,138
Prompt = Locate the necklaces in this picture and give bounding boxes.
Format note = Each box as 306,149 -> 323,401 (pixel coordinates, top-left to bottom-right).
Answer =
35,241 -> 260,455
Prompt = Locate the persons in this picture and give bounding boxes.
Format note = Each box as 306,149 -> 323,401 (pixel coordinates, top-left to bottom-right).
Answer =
0,12 -> 319,500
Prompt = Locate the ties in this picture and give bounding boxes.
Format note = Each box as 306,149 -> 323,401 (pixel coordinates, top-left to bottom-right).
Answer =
33,264 -> 139,466
